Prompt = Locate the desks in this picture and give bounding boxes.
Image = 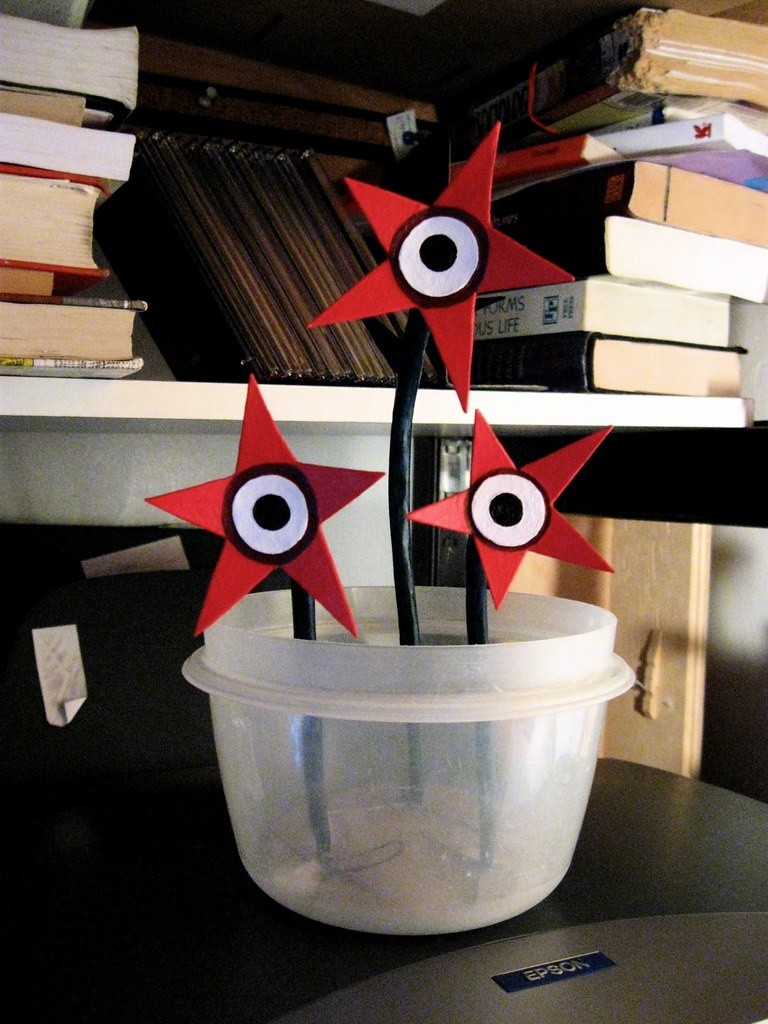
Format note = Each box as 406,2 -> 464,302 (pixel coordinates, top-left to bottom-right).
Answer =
1,736 -> 767,1024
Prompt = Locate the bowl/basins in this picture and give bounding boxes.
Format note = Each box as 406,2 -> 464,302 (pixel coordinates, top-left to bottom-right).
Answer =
177,585 -> 636,936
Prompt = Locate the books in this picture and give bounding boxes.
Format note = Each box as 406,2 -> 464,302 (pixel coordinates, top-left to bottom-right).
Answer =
445,7 -> 768,396
0,15 -> 149,379
